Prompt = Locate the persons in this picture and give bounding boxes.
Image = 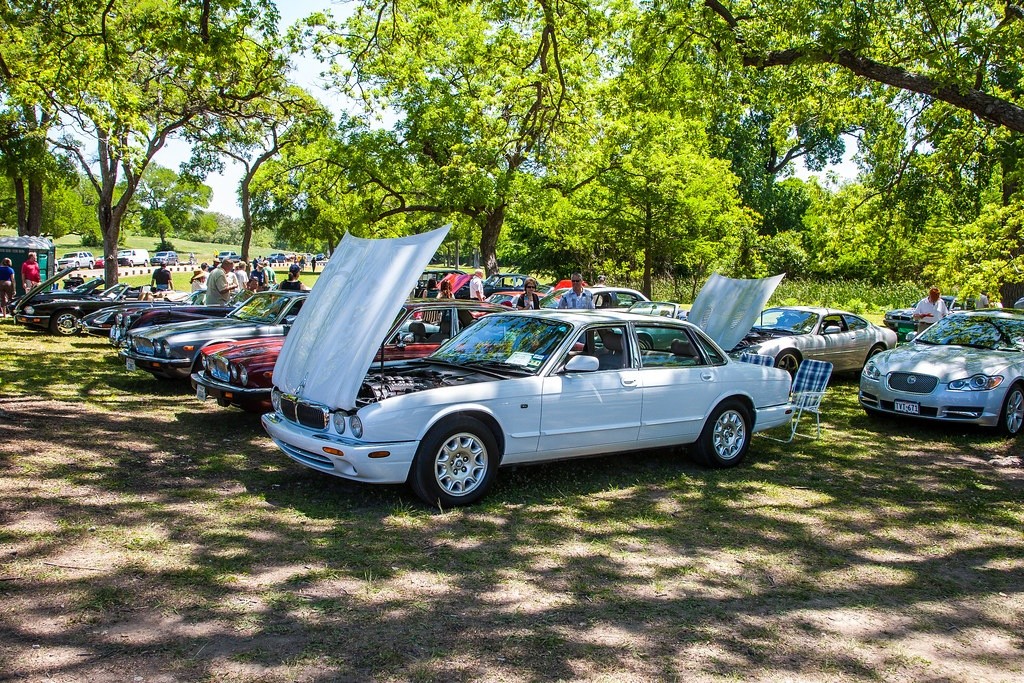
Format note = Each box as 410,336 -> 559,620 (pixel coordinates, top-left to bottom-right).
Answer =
0,258 -> 17,318
976,291 -> 990,308
407,270 -> 487,301
913,288 -> 949,334
516,278 -> 541,310
558,274 -> 607,342
21,254 -> 41,292
151,262 -> 173,290
188,250 -> 316,305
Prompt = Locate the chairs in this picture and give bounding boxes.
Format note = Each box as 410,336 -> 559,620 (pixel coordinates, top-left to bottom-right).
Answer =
823,319 -> 839,331
145,292 -> 153,301
597,332 -> 626,370
138,292 -> 146,301
757,357 -> 835,442
425,316 -> 451,343
602,294 -> 611,307
408,322 -> 429,343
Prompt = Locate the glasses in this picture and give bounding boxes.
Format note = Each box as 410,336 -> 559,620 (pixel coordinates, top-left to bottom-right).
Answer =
572,279 -> 581,282
526,286 -> 535,288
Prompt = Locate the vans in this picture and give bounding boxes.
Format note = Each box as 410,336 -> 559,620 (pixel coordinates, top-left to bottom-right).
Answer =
116,249 -> 149,268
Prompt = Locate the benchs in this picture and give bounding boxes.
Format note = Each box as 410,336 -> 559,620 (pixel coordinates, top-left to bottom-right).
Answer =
593,337 -> 701,368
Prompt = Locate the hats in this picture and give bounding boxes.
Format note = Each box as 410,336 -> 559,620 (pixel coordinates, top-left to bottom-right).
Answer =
290,265 -> 302,273
257,262 -> 264,267
192,270 -> 205,279
930,289 -> 940,295
239,261 -> 248,266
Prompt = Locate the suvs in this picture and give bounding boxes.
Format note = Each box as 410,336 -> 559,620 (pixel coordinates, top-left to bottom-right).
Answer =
58,252 -> 95,271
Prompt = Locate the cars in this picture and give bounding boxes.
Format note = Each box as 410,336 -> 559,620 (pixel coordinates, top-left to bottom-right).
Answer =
627,299 -> 690,322
316,254 -> 324,260
8,279 -> 106,315
218,251 -> 240,263
539,286 -> 674,317
415,270 -> 572,310
95,256 -> 105,268
149,251 -> 178,267
11,267 -> 179,338
727,306 -> 899,381
190,298 -> 512,414
118,290 -> 311,397
883,295 -> 973,335
77,290 -> 238,342
265,253 -> 296,263
456,273 -> 539,301
857,309 -> 1024,436
259,224 -> 798,507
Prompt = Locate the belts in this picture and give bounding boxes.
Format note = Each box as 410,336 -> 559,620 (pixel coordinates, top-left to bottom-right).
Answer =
920,322 -> 934,325
